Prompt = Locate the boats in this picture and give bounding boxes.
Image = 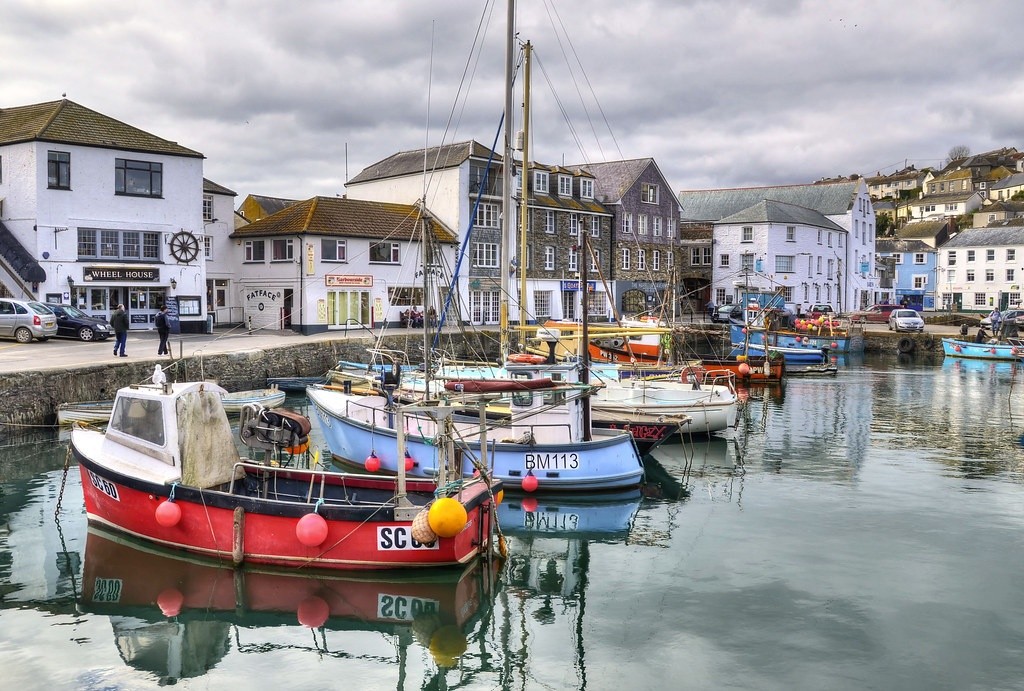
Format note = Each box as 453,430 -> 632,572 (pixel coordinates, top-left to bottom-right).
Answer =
728,316 -> 852,360
587,317 -> 785,384
221,384 -> 286,415
68,363 -> 505,571
941,336 -> 1024,363
77,520 -> 505,668
59,401 -> 115,427
264,376 -> 329,390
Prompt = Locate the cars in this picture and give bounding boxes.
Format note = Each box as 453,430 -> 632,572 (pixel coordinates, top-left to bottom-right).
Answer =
0,298 -> 58,344
980,309 -> 1024,331
851,305 -> 905,323
15,302 -> 116,342
889,309 -> 924,332
804,303 -> 837,318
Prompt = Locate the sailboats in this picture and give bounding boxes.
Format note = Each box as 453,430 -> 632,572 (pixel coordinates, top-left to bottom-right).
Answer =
306,0 -> 741,490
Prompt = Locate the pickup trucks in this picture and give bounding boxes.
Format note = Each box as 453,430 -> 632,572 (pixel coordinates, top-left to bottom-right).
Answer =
710,300 -> 798,325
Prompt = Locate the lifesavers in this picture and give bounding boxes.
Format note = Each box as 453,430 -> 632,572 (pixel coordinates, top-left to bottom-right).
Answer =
508,353 -> 547,362
898,338 -> 914,353
897,354 -> 913,367
925,339 -> 933,349
681,366 -> 702,384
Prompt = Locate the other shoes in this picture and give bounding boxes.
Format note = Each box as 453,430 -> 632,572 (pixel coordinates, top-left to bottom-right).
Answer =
114,350 -> 117,355
120,355 -> 128,357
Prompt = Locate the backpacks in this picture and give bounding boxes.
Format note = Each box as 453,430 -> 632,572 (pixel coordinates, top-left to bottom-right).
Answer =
155,313 -> 167,327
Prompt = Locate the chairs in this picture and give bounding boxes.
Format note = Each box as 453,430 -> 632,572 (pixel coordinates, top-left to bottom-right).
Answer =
824,308 -> 829,311
815,308 -> 818,311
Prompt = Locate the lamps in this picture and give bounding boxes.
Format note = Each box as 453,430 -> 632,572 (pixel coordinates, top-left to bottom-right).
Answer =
170,278 -> 177,289
67,277 -> 74,287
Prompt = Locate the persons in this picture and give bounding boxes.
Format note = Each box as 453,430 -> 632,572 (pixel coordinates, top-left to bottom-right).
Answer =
989,307 -> 1002,336
884,299 -> 889,304
156,305 -> 171,356
402,306 -> 437,328
110,304 -> 129,356
900,298 -> 905,305
706,300 -> 715,316
976,325 -> 992,343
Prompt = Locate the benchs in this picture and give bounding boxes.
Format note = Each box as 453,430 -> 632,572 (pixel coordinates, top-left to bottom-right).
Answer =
400,310 -> 438,327
